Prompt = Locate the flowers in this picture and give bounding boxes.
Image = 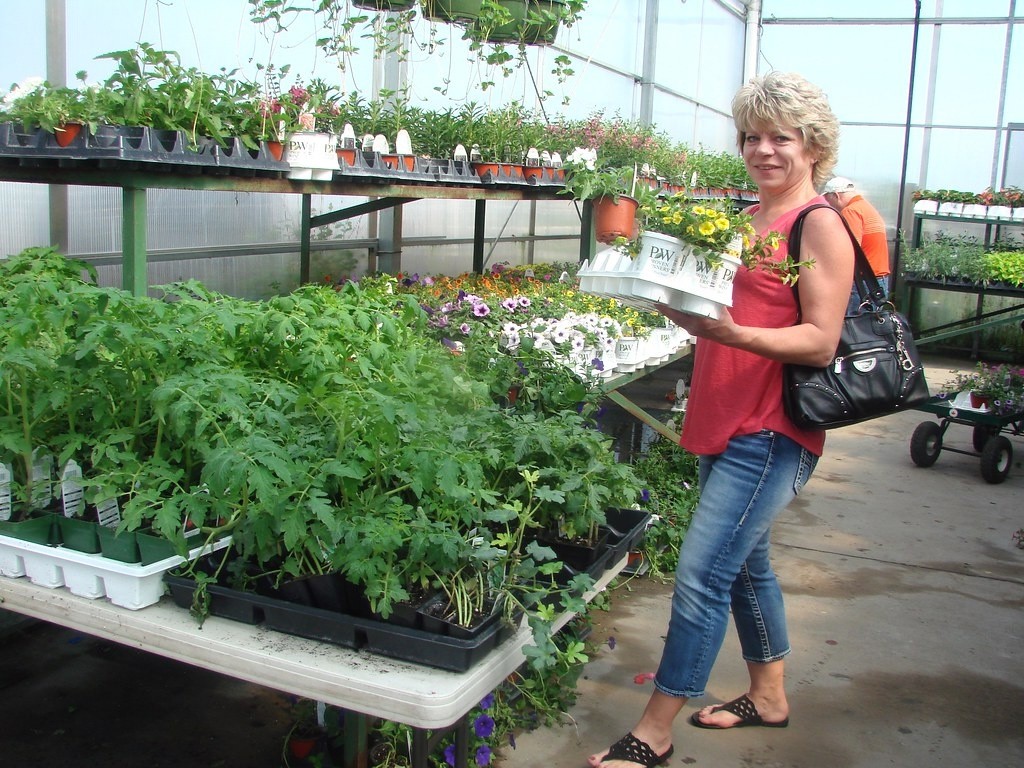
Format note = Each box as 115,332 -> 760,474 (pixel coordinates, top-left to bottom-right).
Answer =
912,185 -> 1024,207
937,363 -> 1024,416
338,197 -> 815,365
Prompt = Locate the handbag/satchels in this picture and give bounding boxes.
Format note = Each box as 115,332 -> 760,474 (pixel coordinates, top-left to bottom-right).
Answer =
781,204 -> 930,429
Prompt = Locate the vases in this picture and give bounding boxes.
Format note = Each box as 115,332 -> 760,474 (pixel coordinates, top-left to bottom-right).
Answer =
689,250 -> 744,298
983,394 -> 991,408
589,349 -> 612,377
616,338 -> 638,372
635,234 -> 684,280
658,329 -> 671,362
970,392 -> 982,408
914,200 -> 1024,222
646,329 -> 661,366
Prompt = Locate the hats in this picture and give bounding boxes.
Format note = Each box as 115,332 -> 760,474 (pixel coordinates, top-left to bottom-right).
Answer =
819,176 -> 855,195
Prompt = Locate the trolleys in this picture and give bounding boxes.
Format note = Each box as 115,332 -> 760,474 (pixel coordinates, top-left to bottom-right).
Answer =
910,391 -> 1024,486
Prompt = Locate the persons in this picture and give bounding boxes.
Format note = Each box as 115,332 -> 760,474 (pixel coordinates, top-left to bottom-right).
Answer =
821,176 -> 890,318
584,72 -> 856,768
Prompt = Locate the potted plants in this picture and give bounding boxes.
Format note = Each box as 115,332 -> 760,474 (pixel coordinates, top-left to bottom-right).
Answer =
0,248 -> 641,726
0,44 -> 758,245
899,228 -> 1024,288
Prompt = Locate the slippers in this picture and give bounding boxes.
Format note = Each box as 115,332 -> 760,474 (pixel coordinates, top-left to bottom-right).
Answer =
601,731 -> 674,768
691,694 -> 790,727
